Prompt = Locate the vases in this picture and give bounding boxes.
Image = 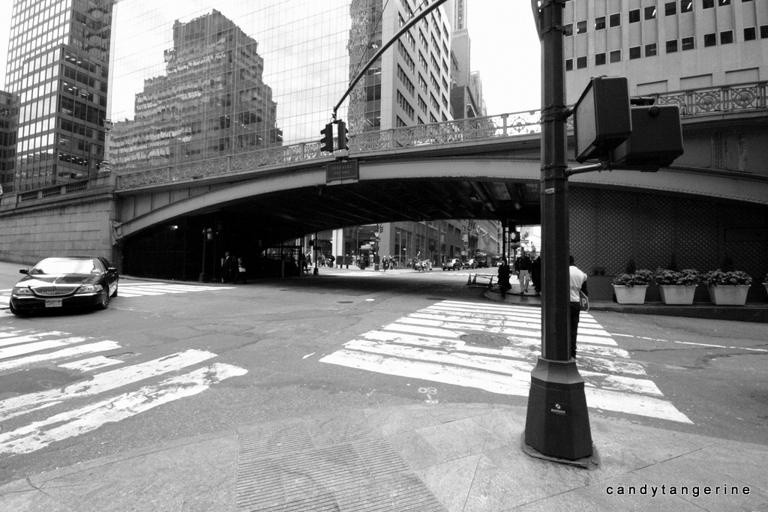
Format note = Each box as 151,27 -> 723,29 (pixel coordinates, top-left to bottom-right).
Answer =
611,283 -> 768,306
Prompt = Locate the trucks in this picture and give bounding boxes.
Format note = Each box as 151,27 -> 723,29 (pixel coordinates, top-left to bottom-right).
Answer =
473,251 -> 502,268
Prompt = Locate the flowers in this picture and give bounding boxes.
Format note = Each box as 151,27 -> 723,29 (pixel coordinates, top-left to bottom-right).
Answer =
612,269 -> 768,286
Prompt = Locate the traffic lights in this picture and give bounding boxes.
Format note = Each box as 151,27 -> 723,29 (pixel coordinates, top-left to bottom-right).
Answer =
338,121 -> 349,151
574,76 -> 632,160
320,123 -> 334,153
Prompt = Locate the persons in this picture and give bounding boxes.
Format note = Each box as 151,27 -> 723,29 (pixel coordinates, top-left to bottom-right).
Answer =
568,253 -> 590,362
309,248 -> 436,275
515,251 -> 533,299
496,256 -> 512,304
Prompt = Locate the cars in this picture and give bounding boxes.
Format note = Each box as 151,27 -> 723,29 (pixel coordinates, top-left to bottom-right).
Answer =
462,258 -> 478,269
10,253 -> 120,316
442,258 -> 462,271
